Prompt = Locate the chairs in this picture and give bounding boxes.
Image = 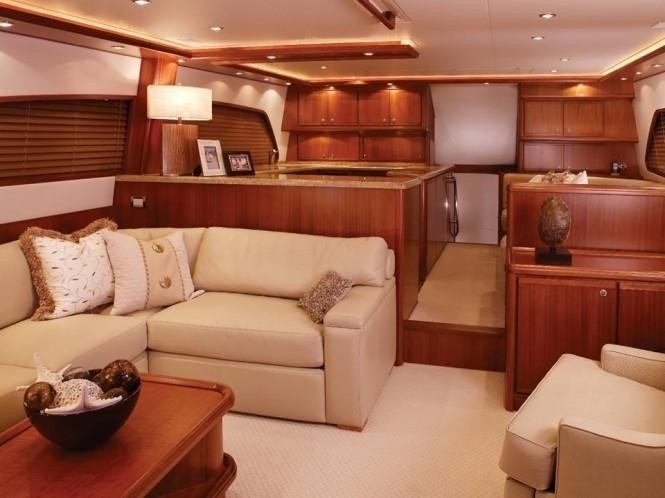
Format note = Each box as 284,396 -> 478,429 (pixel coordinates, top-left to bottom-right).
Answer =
498,343 -> 665,498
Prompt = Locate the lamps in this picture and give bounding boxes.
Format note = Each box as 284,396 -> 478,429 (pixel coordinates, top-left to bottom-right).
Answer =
146,83 -> 212,126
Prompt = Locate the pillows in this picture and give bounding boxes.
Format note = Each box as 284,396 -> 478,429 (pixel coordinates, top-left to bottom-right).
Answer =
19,217 -> 117,320
103,227 -> 208,316
299,267 -> 354,324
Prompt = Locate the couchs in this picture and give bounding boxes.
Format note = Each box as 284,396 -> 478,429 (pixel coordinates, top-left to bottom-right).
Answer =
1,218 -> 398,434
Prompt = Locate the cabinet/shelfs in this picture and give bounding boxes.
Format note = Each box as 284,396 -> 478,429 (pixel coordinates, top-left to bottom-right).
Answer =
294,130 -> 361,163
297,86 -> 358,128
523,101 -> 638,139
358,86 -> 435,130
362,129 -> 435,166
519,143 -> 635,172
504,181 -> 664,411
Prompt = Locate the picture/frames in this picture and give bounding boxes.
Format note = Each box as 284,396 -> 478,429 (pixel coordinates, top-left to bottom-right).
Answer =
223,150 -> 257,175
197,137 -> 227,176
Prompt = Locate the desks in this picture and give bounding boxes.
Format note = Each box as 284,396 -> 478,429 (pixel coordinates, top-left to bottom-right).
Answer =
1,372 -> 238,498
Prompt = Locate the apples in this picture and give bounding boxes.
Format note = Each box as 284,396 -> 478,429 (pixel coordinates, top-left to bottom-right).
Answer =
24,358 -> 139,411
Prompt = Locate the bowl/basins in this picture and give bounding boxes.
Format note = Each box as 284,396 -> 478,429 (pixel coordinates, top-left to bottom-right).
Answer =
23,367 -> 142,453
